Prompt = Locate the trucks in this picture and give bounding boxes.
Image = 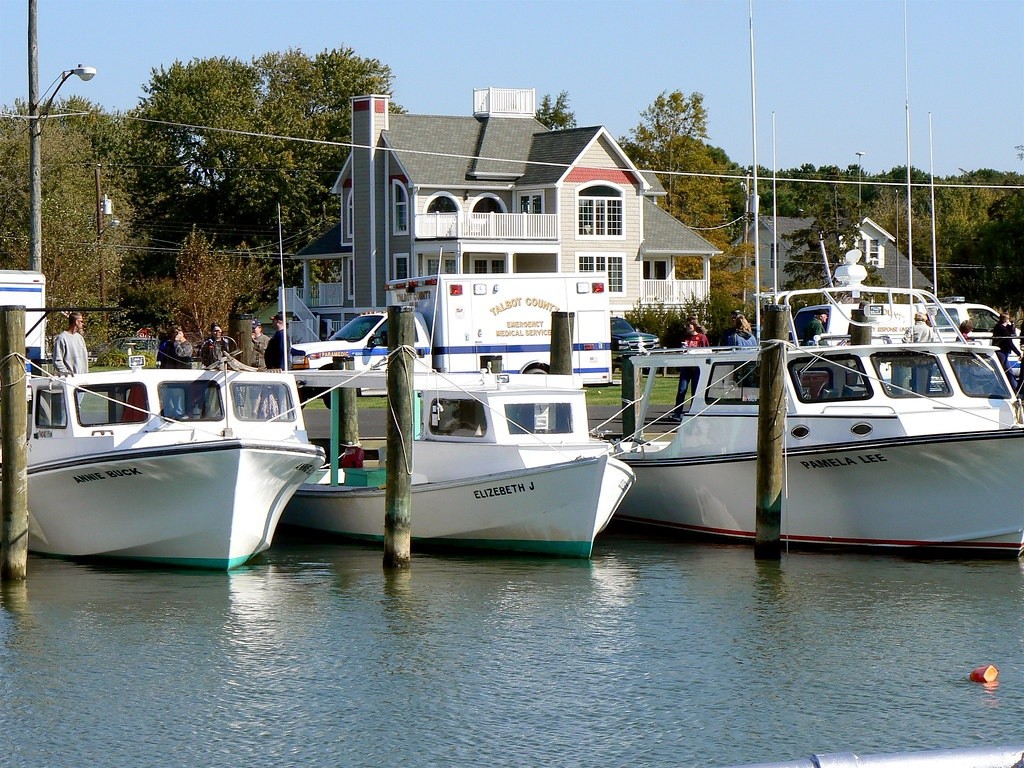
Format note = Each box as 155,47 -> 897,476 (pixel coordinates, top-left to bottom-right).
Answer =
793,295 -> 1023,377
291,272 -> 613,387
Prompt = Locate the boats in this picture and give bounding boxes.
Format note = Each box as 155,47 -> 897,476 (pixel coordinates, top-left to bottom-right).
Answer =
273,365 -> 638,561
590,289 -> 1024,564
0,355 -> 324,571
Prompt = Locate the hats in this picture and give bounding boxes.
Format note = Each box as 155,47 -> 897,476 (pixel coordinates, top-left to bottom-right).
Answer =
252,321 -> 261,329
211,326 -> 221,333
270,314 -> 283,321
815,309 -> 829,315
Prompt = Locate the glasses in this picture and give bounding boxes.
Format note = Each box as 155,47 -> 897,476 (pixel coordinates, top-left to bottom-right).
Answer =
686,324 -> 691,327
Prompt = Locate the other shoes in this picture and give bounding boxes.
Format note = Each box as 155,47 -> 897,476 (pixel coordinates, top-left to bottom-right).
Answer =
668,414 -> 682,422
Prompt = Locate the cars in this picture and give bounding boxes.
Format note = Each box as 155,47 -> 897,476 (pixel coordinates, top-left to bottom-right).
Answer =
99,337 -> 161,365
609,317 -> 661,374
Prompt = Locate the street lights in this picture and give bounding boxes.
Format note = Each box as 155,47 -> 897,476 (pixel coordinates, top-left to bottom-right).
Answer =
28,65 -> 97,271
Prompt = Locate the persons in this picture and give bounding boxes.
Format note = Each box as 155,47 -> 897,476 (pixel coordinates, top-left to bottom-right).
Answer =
724,313 -> 759,388
53,311 -> 89,426
902,312 -> 936,396
802,309 -> 830,346
955,321 -> 975,388
667,316 -> 713,422
156,313 -> 293,421
991,313 -> 1023,400
